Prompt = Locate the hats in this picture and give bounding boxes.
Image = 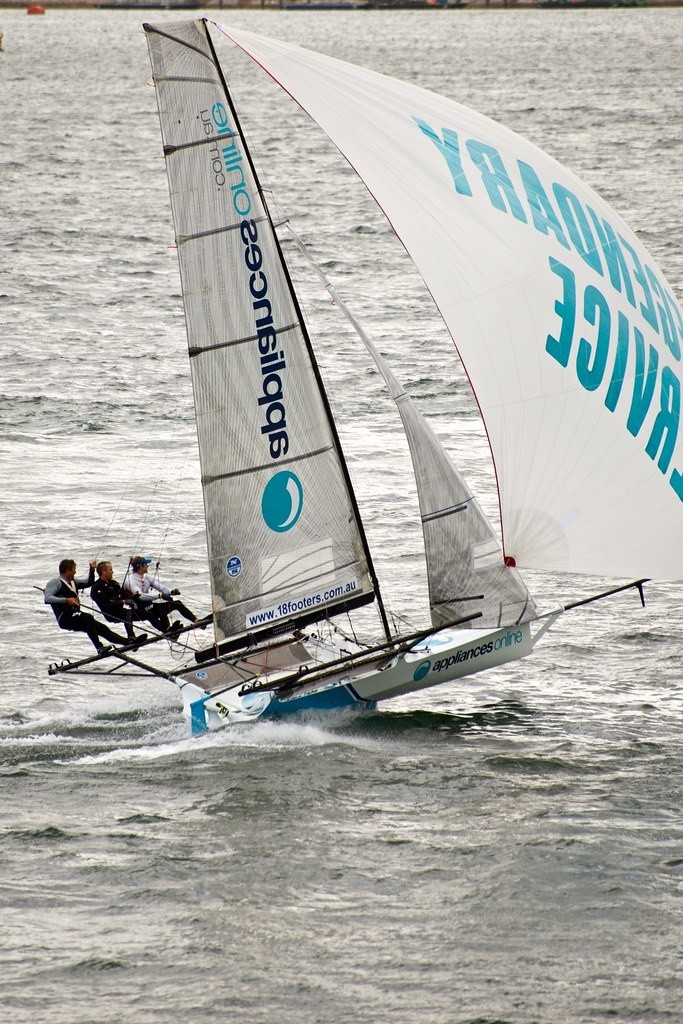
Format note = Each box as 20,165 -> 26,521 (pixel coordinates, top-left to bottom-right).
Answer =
131,556 -> 151,568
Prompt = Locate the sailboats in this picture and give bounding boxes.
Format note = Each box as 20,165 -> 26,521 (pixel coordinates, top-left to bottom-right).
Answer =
45,17 -> 682,739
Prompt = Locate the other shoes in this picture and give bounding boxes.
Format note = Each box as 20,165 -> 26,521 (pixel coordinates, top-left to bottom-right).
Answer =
164,620 -> 183,641
98,646 -> 112,655
131,634 -> 148,652
198,613 -> 213,630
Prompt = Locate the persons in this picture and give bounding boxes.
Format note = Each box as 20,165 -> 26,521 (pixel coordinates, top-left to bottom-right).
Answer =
44,556 -> 211,654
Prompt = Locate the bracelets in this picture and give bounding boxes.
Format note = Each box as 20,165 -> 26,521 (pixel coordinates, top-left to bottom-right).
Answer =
160,592 -> 162,599
